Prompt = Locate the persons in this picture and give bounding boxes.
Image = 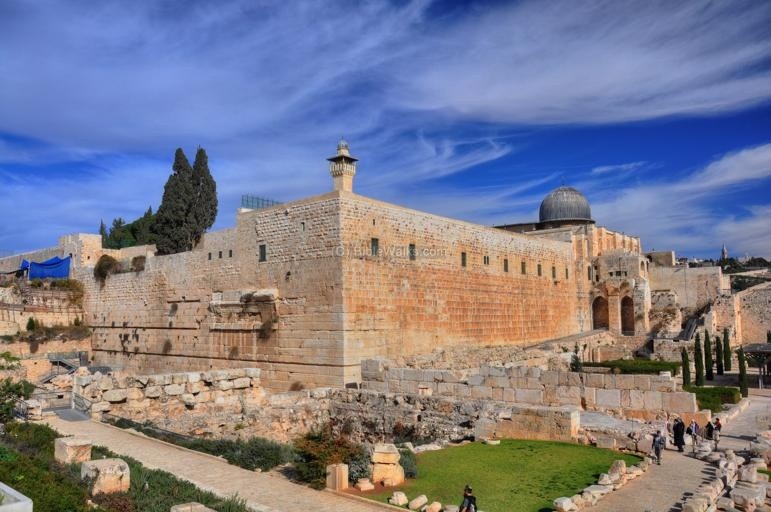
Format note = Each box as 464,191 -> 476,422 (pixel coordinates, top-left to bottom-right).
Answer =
713,417 -> 721,434
457,484 -> 477,512
672,419 -> 685,453
704,421 -> 713,439
713,427 -> 720,452
676,417 -> 685,446
651,431 -> 665,465
688,419 -> 699,455
